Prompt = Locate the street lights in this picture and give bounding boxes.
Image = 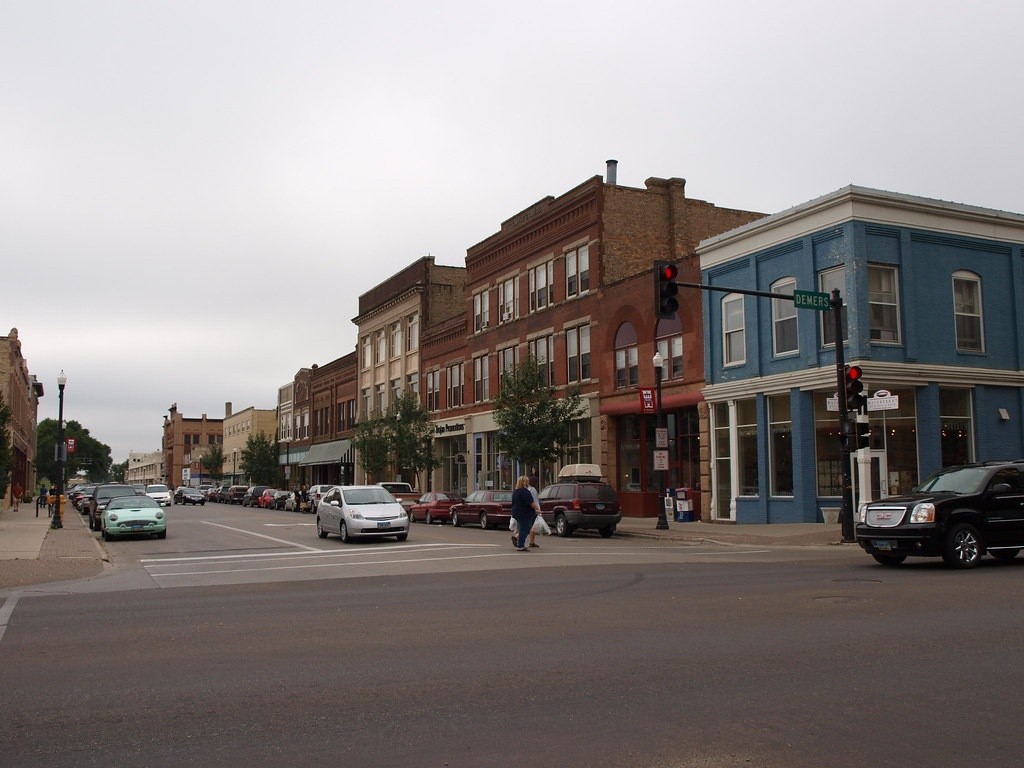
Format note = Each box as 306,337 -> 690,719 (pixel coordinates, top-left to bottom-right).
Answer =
233,448 -> 236,486
199,454 -> 202,487
396,412 -> 401,490
50,369 -> 67,528
286,437 -> 290,490
652,350 -> 669,529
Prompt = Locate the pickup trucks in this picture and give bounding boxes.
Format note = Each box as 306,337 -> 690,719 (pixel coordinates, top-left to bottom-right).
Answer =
375,481 -> 423,513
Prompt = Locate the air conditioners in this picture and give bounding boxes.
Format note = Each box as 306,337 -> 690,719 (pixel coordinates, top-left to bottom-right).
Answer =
481,321 -> 489,329
503,313 -> 510,320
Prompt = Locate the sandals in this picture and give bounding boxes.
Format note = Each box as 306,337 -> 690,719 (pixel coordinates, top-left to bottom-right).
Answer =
529,542 -> 541,548
511,536 -> 518,548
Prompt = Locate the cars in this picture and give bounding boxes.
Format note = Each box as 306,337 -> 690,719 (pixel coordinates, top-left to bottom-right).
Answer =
101,495 -> 167,541
145,484 -> 173,506
88,485 -> 137,531
856,458 -> 1024,569
67,481 -> 147,515
194,485 -> 341,513
316,486 -> 410,543
409,491 -> 464,524
174,488 -> 206,507
450,490 -> 515,529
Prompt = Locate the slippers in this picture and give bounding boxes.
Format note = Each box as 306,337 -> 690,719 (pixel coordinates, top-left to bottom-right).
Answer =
517,547 -> 530,551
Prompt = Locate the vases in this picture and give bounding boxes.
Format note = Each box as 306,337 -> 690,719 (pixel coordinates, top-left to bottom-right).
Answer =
820,507 -> 842,524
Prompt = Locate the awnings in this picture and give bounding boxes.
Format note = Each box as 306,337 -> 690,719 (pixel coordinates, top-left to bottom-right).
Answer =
298,439 -> 355,466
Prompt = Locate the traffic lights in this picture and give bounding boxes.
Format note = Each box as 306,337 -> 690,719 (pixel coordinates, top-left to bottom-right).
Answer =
658,264 -> 678,315
844,365 -> 864,410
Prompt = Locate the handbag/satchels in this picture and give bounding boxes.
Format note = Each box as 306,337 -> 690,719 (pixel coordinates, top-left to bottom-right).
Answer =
530,514 -> 551,536
509,518 -> 519,534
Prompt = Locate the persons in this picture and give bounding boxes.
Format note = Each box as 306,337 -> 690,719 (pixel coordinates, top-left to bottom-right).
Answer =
511,476 -> 543,551
49,485 -> 55,496
294,485 -> 311,514
13,482 -> 23,512
40,484 -> 47,509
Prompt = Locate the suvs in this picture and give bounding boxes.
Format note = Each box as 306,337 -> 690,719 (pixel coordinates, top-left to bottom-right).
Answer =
538,464 -> 622,538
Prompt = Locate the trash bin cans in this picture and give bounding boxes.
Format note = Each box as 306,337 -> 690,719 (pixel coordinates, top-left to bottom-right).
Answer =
48,495 -> 67,521
675,487 -> 694,523
